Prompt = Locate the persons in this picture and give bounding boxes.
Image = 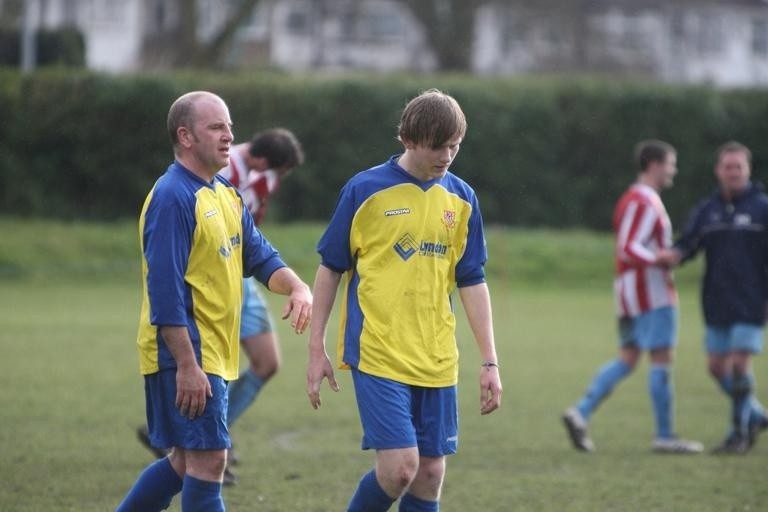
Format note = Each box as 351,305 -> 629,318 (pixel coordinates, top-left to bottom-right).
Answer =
661,139 -> 768,458
302,85 -> 503,511
561,140 -> 705,457
114,88 -> 314,511
132,127 -> 302,487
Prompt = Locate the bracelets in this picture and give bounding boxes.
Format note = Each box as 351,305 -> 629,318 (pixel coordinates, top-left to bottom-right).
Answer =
480,361 -> 500,371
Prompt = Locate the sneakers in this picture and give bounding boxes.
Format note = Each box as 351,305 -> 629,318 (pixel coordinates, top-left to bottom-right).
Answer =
711,411 -> 767,457
562,406 -> 596,452
650,436 -> 708,451
